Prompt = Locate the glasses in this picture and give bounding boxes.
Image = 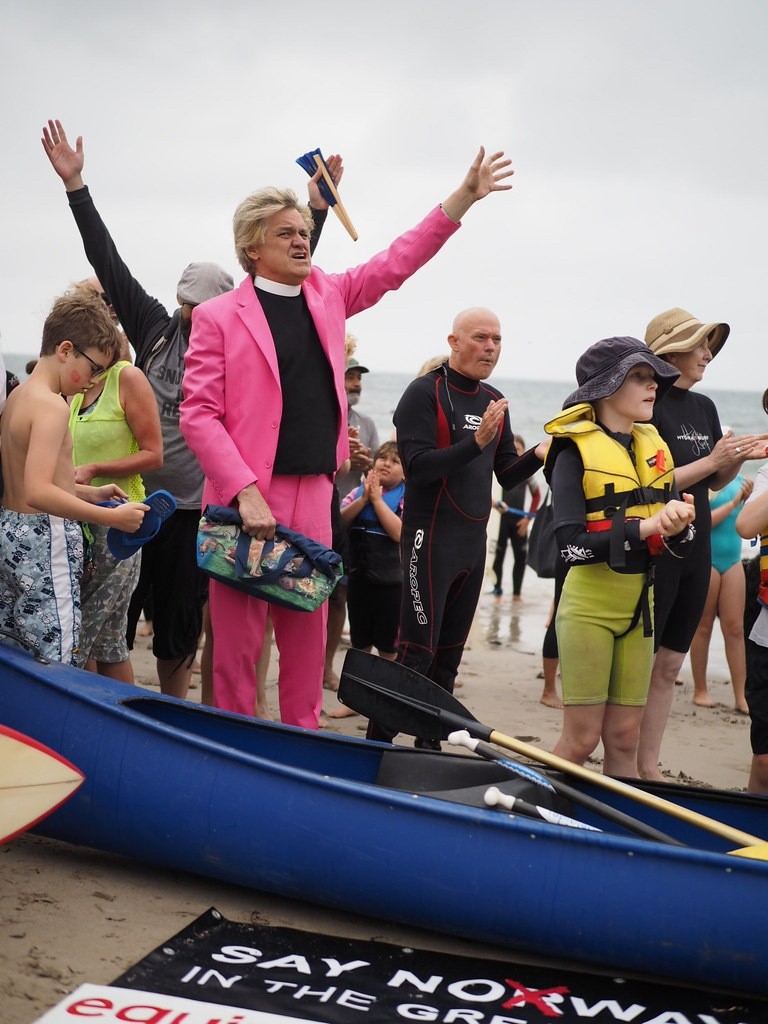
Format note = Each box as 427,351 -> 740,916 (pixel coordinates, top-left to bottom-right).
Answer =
182,301 -> 196,313
73,346 -> 107,378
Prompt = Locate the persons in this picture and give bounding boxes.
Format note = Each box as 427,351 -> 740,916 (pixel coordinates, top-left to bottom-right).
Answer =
179,145 -> 516,731
0,120 -> 346,723
540,307 -> 768,795
321,307 -> 553,751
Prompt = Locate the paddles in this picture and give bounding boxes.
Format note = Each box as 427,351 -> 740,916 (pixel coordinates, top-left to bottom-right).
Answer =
338,647 -> 768,846
485,786 -> 768,858
448,730 -> 689,847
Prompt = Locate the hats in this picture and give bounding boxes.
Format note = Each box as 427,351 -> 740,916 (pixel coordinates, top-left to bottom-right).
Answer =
345,357 -> 369,374
178,261 -> 234,304
562,337 -> 682,410
645,308 -> 730,362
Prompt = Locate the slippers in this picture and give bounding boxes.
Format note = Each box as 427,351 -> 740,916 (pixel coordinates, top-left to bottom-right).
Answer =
97,488 -> 178,561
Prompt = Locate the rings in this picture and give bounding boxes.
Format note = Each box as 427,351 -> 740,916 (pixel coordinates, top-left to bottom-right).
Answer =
736,447 -> 740,453
490,411 -> 494,415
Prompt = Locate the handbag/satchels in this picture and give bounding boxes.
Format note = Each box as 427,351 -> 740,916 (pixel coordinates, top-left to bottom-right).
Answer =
194,503 -> 346,612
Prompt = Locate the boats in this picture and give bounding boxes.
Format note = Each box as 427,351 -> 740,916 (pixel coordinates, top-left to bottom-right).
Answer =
0,630 -> 768,994
